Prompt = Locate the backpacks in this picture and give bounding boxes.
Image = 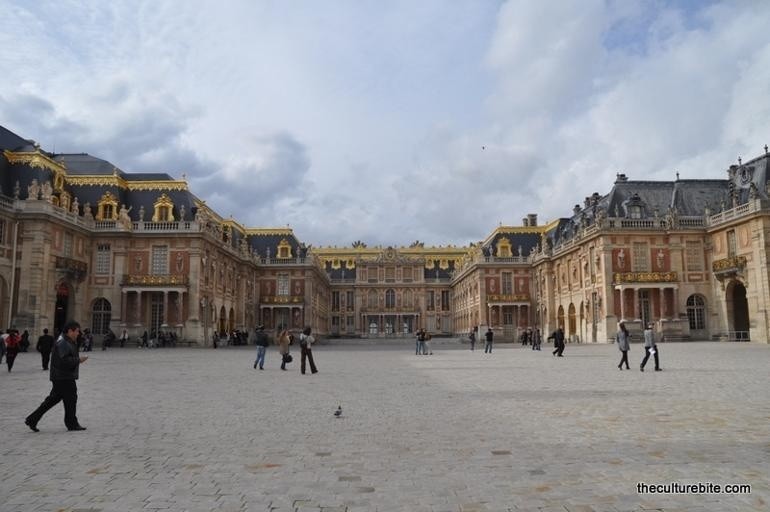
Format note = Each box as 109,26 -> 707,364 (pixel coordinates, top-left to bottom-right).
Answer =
300,336 -> 308,348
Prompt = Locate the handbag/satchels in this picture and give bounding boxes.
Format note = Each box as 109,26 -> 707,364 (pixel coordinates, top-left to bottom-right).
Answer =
283,352 -> 292,362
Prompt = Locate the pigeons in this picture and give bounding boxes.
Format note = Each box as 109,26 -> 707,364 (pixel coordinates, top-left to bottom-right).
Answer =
333,404 -> 342,417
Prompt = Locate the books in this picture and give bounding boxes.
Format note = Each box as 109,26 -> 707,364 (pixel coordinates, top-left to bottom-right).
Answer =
648,347 -> 658,355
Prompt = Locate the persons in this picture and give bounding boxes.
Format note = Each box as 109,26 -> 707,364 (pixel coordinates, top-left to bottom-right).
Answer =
484,328 -> 493,353
0,325 -> 177,372
298,326 -> 319,374
277,328 -> 291,370
520,326 -> 541,350
253,325 -> 270,370
551,325 -> 566,356
25,320 -> 89,433
615,322 -> 633,370
415,328 -> 433,356
210,325 -> 250,349
639,322 -> 662,372
470,330 -> 477,350
